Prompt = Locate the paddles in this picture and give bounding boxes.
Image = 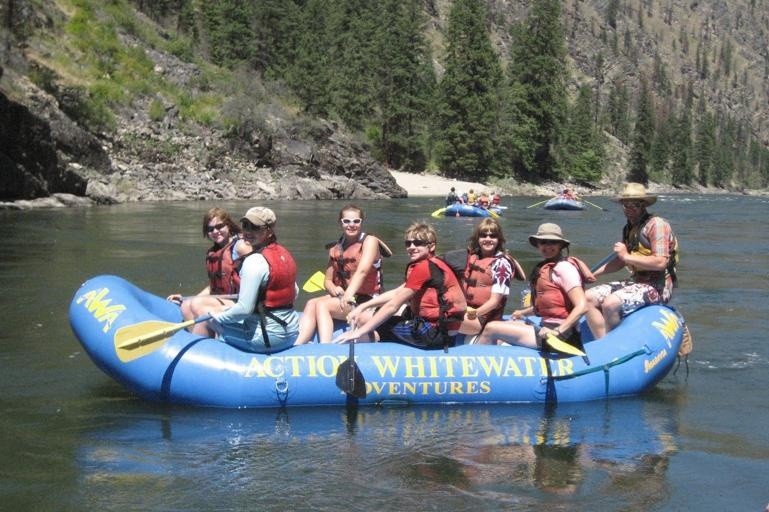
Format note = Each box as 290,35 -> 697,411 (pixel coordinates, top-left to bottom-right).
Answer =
484,206 -> 501,219
336,319 -> 366,399
519,317 -> 587,357
583,201 -> 611,212
303,271 -> 358,307
114,308 -> 232,363
431,205 -> 450,216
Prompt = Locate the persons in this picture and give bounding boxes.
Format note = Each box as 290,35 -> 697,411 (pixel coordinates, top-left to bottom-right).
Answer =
476,223 -> 597,352
192,207 -> 299,353
332,222 -> 467,348
585,182 -> 679,339
292,206 -> 392,346
560,189 -> 580,200
167,206 -> 254,332
445,187 -> 502,210
459,217 -> 527,336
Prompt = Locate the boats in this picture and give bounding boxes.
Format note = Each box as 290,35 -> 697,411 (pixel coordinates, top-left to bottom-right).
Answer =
445,203 -> 502,218
543,198 -> 586,210
67,273 -> 685,410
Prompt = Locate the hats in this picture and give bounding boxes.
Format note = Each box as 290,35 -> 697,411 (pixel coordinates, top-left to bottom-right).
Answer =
609,182 -> 656,207
240,207 -> 277,227
529,223 -> 570,247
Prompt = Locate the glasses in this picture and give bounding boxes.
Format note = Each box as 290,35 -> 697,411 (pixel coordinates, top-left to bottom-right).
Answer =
622,201 -> 641,209
241,221 -> 268,232
207,223 -> 225,232
479,232 -> 497,238
341,218 -> 362,224
405,239 -> 431,246
